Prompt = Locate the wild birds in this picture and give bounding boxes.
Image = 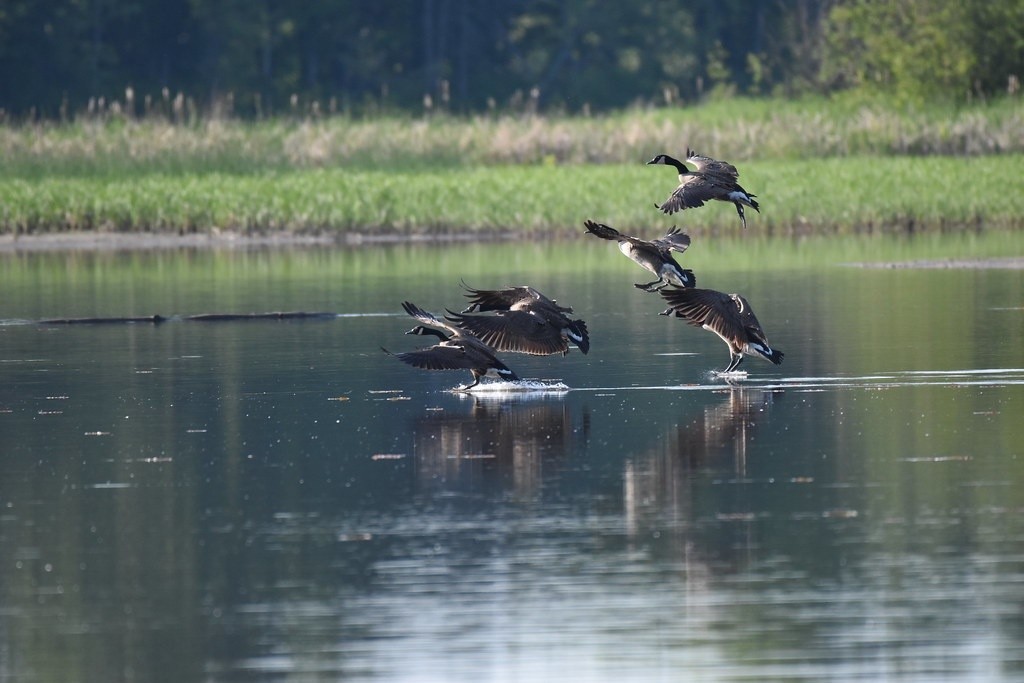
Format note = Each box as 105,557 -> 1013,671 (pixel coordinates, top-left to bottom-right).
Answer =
582,219 -> 785,373
646,147 -> 760,229
379,277 -> 589,390
